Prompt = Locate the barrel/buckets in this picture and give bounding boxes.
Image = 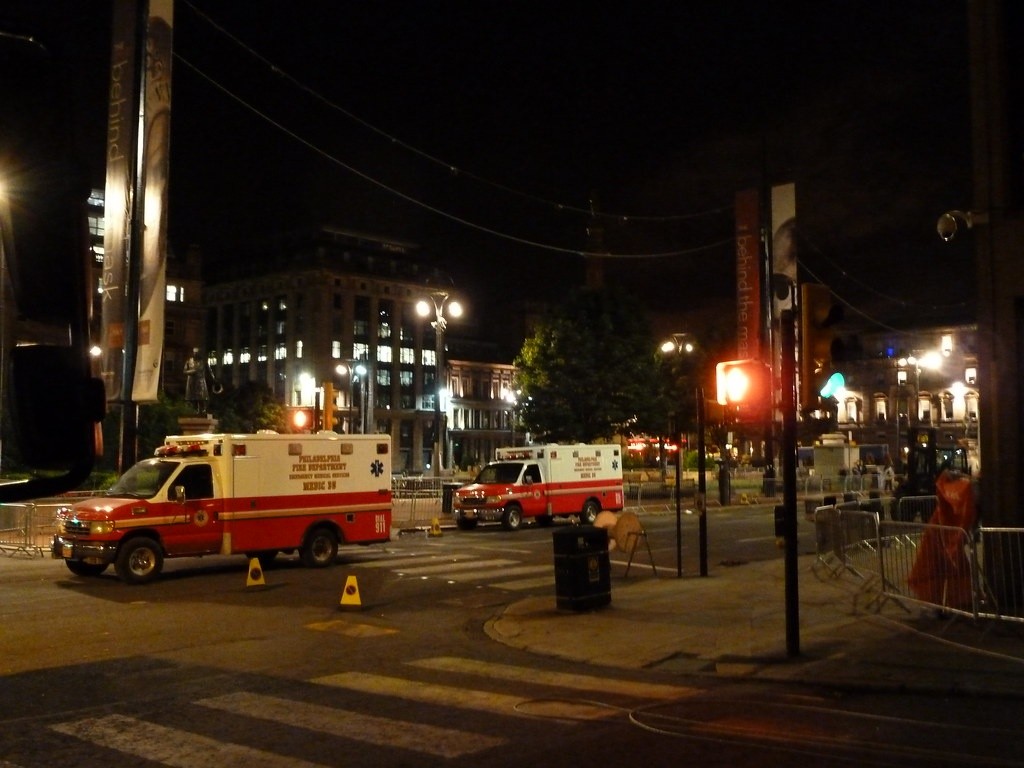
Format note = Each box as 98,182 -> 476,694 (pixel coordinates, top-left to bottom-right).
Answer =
552,525 -> 612,614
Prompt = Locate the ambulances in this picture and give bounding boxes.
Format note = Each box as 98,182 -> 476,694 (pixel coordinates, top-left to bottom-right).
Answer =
50,426 -> 394,585
451,443 -> 627,528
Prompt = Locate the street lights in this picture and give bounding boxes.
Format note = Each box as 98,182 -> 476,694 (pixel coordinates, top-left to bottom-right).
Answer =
415,288 -> 464,494
660,331 -> 710,574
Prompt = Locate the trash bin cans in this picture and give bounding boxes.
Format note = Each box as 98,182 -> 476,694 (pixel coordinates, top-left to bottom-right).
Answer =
551,524 -> 614,615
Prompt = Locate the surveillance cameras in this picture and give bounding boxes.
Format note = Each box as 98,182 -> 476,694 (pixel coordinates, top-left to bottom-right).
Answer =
936,214 -> 957,242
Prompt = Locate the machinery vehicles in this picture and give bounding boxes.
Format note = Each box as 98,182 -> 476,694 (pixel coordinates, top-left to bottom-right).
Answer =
888,426 -> 970,523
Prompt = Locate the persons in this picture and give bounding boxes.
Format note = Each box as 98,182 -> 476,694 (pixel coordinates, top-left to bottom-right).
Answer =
711,448 -> 957,501
